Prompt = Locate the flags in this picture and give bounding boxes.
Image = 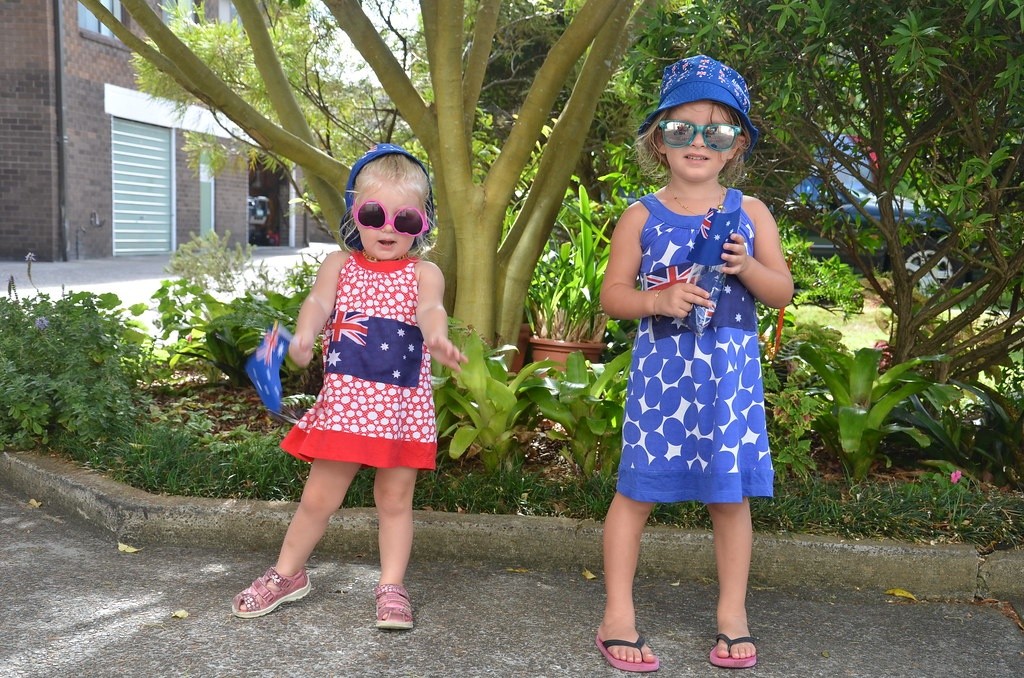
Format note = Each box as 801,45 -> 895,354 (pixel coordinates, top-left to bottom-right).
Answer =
246,322 -> 292,413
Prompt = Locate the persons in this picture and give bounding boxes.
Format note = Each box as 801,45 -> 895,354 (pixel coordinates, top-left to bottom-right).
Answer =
594,56 -> 793,670
232,143 -> 469,628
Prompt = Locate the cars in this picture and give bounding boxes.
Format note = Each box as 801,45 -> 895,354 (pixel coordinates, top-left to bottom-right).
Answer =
767,165 -> 987,292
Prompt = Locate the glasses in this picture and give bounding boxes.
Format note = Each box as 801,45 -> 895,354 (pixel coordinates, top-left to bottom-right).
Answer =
658,120 -> 743,151
352,199 -> 429,238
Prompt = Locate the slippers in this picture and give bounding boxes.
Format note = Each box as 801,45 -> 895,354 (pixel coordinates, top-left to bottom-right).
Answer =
595,632 -> 660,673
709,633 -> 758,669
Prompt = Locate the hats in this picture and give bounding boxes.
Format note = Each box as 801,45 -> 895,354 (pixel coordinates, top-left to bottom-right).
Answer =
637,54 -> 759,165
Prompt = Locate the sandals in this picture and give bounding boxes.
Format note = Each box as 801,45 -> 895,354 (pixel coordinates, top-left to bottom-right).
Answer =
373,582 -> 415,632
231,565 -> 312,619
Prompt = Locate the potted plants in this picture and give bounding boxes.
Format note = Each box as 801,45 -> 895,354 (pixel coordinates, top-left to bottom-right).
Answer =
497,206 -> 530,374
524,184 -> 611,378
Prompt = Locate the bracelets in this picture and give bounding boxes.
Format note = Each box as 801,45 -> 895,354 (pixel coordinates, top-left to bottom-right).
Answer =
654,291 -> 662,321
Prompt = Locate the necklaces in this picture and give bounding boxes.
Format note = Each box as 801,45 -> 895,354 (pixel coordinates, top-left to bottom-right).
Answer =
363,251 -> 408,260
668,179 -> 724,216
